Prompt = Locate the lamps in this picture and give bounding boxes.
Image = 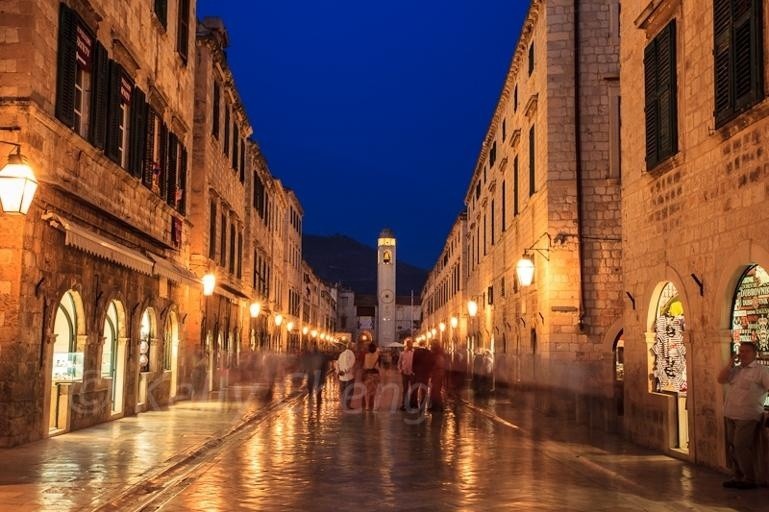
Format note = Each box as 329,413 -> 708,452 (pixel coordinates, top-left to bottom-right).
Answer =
516,244 -> 551,287
0,139 -> 39,216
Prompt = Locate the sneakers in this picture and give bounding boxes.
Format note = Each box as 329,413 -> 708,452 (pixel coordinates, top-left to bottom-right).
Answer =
722,479 -> 756,489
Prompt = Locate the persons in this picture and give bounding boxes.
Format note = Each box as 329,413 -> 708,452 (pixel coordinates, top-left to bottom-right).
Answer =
305,339 -> 454,412
716,342 -> 769,490
